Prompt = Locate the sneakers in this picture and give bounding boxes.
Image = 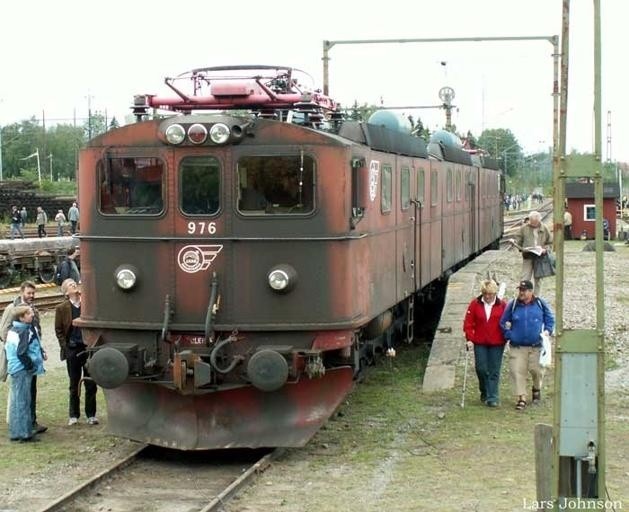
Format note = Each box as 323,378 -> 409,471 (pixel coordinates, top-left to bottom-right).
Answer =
11,417 -> 98,441
488,401 -> 496,407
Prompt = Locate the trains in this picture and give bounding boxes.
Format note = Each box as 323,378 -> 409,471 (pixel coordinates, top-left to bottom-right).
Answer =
70,64 -> 505,457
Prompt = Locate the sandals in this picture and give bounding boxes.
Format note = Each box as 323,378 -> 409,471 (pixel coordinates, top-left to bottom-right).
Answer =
532,386 -> 540,404
516,400 -> 527,410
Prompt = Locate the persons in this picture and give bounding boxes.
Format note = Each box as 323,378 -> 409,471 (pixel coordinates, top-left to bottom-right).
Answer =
499,280 -> 555,410
4,305 -> 44,440
563,207 -> 572,239
462,279 -> 512,407
9,201 -> 79,238
0,282 -> 48,434
504,191 -> 544,211
514,211 -> 553,298
61,245 -> 80,286
55,278 -> 102,425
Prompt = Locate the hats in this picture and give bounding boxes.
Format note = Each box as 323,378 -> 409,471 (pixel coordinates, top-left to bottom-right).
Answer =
517,280 -> 532,289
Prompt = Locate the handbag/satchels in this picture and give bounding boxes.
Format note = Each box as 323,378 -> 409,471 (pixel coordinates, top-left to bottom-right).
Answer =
534,248 -> 553,278
